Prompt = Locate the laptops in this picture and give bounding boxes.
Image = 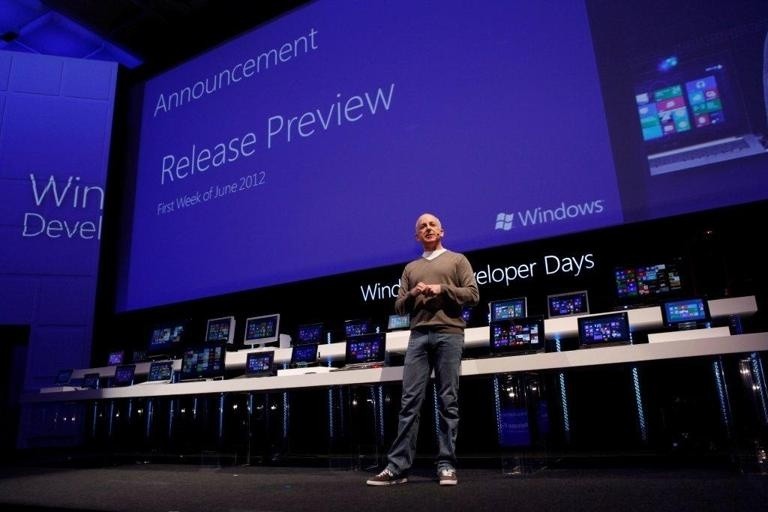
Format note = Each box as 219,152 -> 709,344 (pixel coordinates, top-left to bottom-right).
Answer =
628,49 -> 768,177
330,333 -> 386,372
578,312 -> 631,349
483,315 -> 544,359
547,290 -> 590,318
611,261 -> 672,310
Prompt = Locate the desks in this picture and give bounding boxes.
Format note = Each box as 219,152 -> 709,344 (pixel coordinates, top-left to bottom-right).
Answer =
20,294 -> 768,470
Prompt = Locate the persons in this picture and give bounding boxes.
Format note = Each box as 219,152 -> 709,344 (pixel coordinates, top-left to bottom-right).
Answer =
365,213 -> 482,485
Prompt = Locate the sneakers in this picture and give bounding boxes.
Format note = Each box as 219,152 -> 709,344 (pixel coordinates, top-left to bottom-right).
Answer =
438,468 -> 458,486
366,468 -> 408,485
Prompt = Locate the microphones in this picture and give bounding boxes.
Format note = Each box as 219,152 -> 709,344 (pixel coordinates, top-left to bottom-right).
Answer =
437,230 -> 444,236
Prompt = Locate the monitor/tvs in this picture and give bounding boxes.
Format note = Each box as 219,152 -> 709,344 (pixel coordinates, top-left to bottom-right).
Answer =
244,314 -> 280,348
298,324 -> 322,344
386,313 -> 410,330
345,320 -> 368,341
491,296 -> 528,321
462,307 -> 472,322
55,315 -> 237,388
235,351 -> 274,379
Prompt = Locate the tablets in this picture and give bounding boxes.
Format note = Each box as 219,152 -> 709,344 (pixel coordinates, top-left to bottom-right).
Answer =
660,295 -> 712,327
291,344 -> 318,363
